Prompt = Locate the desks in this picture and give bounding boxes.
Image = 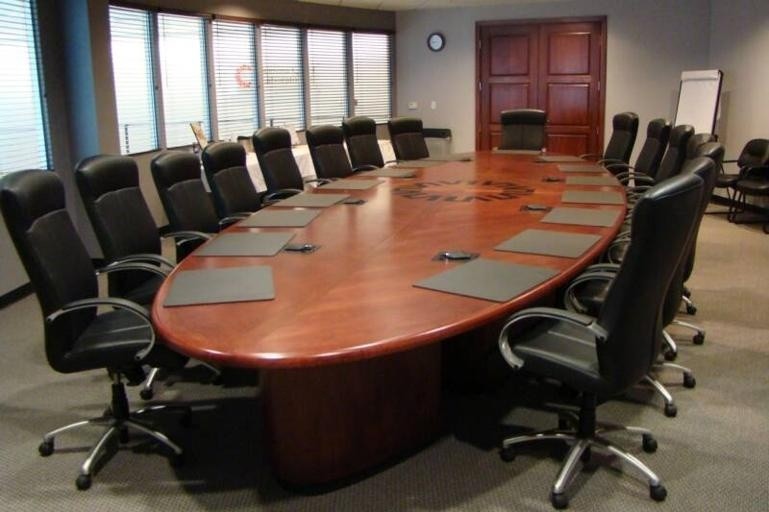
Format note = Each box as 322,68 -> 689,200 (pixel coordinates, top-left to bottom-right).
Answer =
153,149 -> 626,490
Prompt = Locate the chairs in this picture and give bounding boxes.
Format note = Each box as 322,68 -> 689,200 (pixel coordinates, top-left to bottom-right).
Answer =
714,139 -> 769,222
1,170 -> 190,490
73,155 -> 227,400
732,156 -> 769,234
492,108 -> 548,152
498,176 -> 703,509
